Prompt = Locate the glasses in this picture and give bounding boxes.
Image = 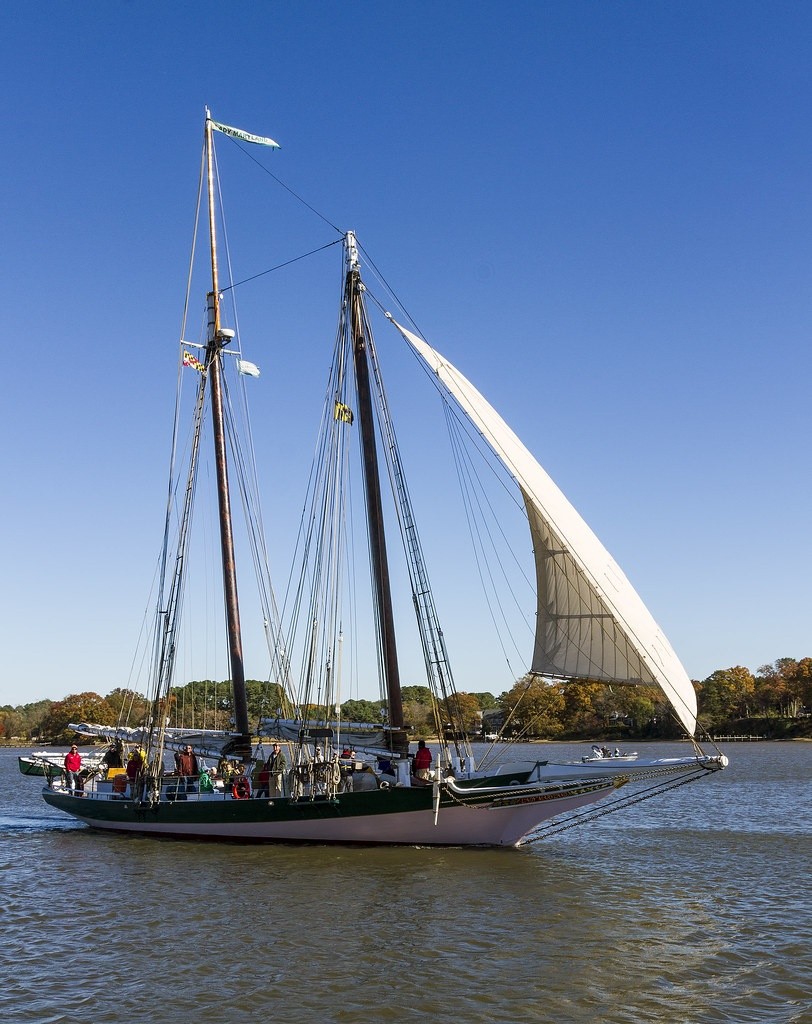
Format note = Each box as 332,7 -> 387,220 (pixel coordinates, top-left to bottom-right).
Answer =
72,749 -> 77,750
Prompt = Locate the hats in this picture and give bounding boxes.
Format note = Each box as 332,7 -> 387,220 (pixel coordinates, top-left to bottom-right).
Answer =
72,745 -> 77,748
272,742 -> 280,745
109,744 -> 115,748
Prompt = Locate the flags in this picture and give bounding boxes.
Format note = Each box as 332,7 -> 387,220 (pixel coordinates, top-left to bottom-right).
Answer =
335,402 -> 353,425
235,359 -> 260,379
212,120 -> 282,151
182,350 -> 207,378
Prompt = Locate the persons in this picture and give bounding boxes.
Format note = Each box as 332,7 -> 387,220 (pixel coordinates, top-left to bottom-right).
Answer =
591,744 -> 621,758
414,741 -> 433,781
64,734 -> 393,802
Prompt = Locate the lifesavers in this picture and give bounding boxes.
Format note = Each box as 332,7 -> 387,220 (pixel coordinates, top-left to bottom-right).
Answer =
233,778 -> 250,799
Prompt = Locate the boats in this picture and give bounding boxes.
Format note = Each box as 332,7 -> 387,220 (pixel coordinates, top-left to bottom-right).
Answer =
581,752 -> 639,763
18,746 -> 108,777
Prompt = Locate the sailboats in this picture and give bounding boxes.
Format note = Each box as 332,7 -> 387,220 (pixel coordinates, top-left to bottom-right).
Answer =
43,106 -> 740,841
